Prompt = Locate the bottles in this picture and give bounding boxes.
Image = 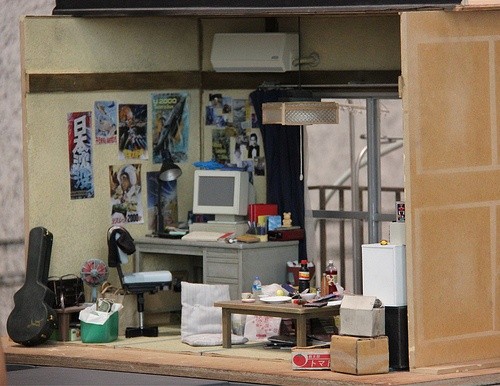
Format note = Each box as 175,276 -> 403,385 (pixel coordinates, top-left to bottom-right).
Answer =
321,259 -> 337,297
299,260 -> 310,293
252,277 -> 261,296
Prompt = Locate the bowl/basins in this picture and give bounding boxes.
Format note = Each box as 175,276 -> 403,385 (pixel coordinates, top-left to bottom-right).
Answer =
298,293 -> 316,300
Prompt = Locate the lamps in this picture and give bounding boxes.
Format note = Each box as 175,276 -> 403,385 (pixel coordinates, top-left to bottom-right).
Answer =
155,149 -> 182,238
263,100 -> 341,126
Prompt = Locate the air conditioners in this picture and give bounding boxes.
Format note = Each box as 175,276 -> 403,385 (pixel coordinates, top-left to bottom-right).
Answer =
210,33 -> 300,73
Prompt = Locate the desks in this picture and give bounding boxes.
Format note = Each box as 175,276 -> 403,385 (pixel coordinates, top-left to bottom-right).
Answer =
214,300 -> 341,349
131,237 -> 299,299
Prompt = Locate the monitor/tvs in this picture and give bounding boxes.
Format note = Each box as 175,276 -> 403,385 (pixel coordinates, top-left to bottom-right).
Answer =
193,170 -> 248,225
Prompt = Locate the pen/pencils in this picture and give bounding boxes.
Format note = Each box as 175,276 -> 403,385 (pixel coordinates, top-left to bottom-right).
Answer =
247,220 -> 258,234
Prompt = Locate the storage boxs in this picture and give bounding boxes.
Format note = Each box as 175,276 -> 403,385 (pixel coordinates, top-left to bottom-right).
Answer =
386,306 -> 408,369
248,204 -> 278,222
290,344 -> 331,371
47,278 -> 85,306
81,311 -> 119,343
275,229 -> 304,241
331,335 -> 390,376
361,243 -> 407,306
340,295 -> 387,337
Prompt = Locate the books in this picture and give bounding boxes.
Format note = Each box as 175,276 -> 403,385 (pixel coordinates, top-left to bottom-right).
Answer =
182,229 -> 236,242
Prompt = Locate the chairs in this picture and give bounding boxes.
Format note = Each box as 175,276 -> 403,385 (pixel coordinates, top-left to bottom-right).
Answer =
106,225 -> 174,338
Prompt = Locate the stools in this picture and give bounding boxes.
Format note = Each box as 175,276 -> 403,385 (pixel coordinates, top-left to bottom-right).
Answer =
55,305 -> 86,342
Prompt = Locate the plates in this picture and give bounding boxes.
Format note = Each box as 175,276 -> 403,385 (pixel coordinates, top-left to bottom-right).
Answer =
260,296 -> 293,305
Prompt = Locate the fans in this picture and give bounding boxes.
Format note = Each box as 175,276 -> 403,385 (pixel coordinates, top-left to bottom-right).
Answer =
80,259 -> 109,306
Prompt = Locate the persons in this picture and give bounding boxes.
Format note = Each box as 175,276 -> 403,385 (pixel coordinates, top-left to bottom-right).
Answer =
111,164 -> 142,224
236,132 -> 265,168
